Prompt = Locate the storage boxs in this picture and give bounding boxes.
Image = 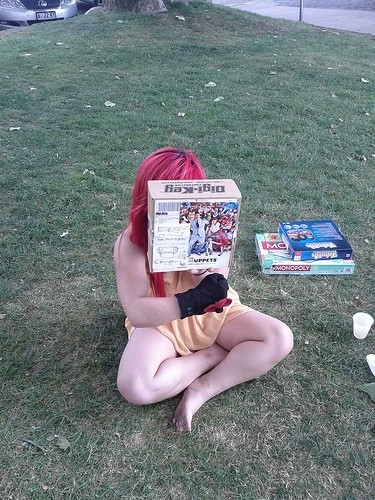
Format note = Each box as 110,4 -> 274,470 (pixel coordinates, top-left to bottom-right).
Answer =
254,232 -> 355,274
145,178 -> 242,274
278,220 -> 353,262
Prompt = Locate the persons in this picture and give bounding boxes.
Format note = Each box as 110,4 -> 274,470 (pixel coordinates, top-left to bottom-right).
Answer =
117,147 -> 296,433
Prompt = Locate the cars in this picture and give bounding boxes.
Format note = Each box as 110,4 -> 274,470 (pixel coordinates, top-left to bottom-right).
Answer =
0,0 -> 78,32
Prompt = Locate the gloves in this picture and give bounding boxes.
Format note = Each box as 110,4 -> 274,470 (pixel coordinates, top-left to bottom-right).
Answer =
175,273 -> 228,319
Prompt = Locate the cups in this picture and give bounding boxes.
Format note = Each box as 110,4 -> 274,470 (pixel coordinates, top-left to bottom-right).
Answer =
352,312 -> 374,339
